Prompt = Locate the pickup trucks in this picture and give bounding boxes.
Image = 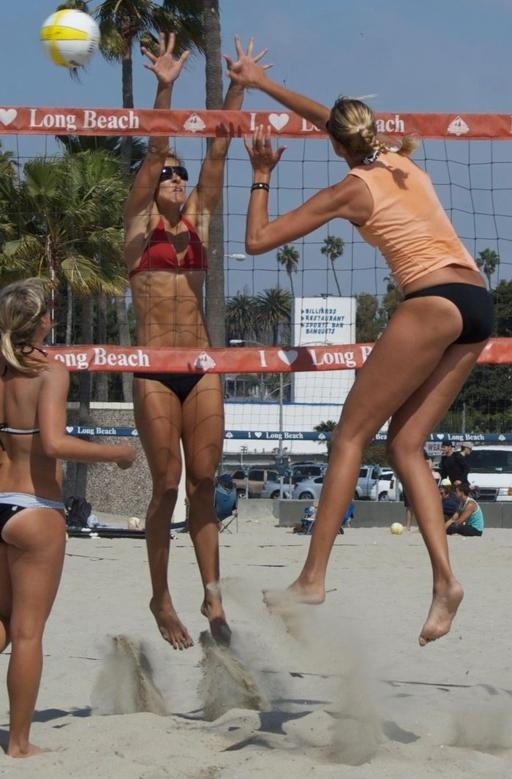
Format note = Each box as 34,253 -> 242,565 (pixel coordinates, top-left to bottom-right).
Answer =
357,466 -> 378,498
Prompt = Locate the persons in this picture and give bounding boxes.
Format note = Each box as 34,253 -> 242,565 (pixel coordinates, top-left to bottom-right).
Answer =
0,276 -> 137,761
438,440 -> 469,485
404,448 -> 432,530
445,482 -> 484,536
226,54 -> 492,648
459,442 -> 472,458
438,485 -> 458,520
127,31 -> 277,651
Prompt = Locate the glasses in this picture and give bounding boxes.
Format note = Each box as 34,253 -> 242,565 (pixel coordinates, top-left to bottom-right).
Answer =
158,165 -> 189,184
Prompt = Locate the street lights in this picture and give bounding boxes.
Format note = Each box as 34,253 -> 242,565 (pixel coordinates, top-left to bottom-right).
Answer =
231,339 -> 285,457
226,250 -> 245,262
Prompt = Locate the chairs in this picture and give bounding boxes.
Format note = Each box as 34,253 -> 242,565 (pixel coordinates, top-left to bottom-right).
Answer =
298,498 -> 353,534
190,483 -> 241,537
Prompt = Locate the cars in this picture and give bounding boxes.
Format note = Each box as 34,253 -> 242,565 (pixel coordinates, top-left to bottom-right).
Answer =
262,474 -> 320,499
293,475 -> 363,500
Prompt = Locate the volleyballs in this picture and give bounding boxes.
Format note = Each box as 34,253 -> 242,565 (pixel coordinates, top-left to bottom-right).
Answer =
391,521 -> 405,536
39,7 -> 100,69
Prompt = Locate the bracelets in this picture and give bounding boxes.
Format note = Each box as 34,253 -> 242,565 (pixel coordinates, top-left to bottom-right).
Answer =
251,183 -> 269,192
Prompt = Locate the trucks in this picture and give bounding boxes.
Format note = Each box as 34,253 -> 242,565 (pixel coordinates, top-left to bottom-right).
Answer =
370,471 -> 406,501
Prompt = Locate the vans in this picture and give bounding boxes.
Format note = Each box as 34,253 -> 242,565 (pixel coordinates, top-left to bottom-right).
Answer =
284,464 -> 323,477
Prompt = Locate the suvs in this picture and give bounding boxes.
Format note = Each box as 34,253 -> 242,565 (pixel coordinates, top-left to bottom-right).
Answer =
439,445 -> 512,502
230,469 -> 279,498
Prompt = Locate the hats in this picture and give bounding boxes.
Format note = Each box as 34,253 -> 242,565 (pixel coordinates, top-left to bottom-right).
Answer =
461,442 -> 473,448
